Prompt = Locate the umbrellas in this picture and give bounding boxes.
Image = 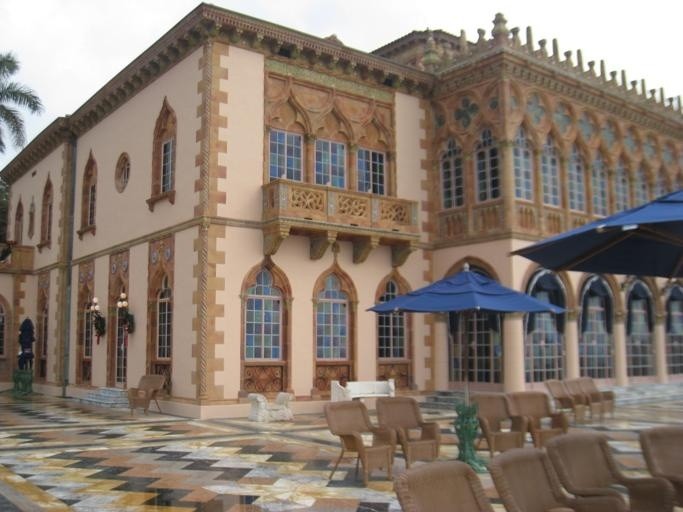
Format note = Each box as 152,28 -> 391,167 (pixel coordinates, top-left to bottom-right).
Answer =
361,261 -> 576,410
14,315 -> 35,373
508,185 -> 683,281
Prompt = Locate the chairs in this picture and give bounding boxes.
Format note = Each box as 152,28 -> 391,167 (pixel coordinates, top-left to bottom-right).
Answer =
126,375 -> 166,416
